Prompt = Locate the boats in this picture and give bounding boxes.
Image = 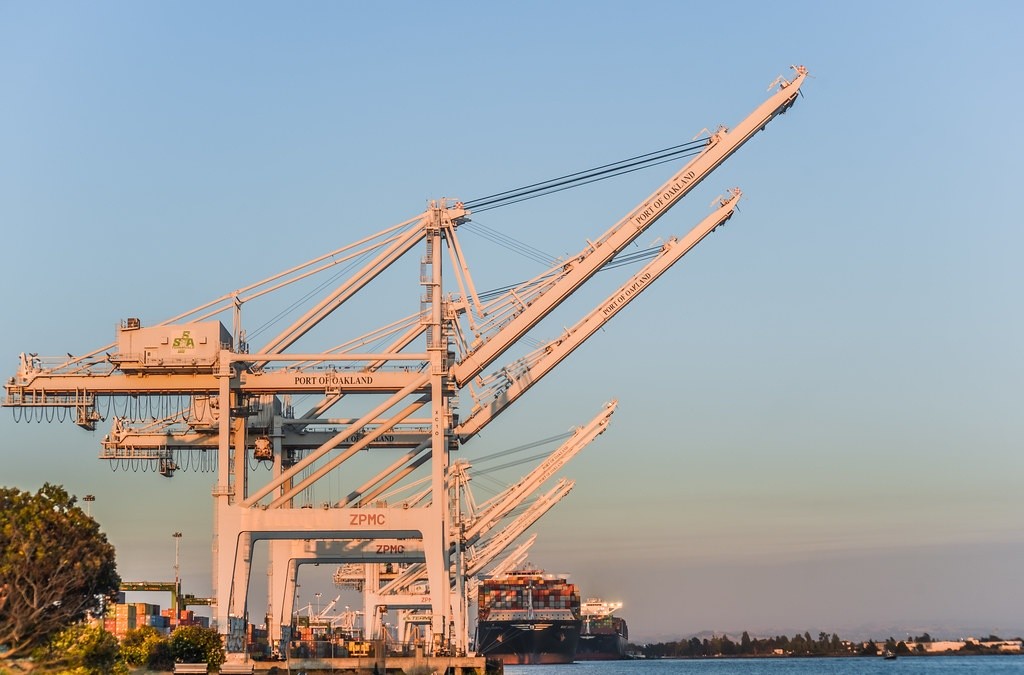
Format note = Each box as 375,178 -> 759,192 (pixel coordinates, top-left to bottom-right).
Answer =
572,596 -> 628,662
884,652 -> 897,661
476,579 -> 584,666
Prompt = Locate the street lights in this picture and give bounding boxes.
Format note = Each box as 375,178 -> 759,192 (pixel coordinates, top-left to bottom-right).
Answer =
82,494 -> 97,518
172,530 -> 182,629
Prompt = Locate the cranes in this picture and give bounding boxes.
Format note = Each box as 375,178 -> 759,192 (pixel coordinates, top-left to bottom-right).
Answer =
0,57 -> 810,675
